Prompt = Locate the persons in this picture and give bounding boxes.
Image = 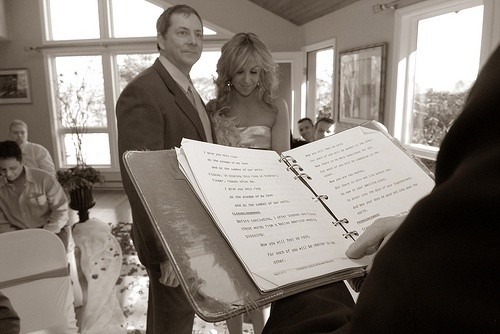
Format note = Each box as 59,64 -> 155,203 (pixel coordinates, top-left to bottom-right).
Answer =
0,140 -> 69,257
258,42 -> 499,333
113,4 -> 214,334
7,120 -> 56,177
290,117 -> 314,148
206,33 -> 289,334
312,117 -> 335,140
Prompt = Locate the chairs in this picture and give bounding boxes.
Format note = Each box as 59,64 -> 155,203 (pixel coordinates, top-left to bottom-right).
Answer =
0,229 -> 79,334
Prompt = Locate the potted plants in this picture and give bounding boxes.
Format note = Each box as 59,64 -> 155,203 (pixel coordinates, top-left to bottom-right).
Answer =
56,72 -> 106,222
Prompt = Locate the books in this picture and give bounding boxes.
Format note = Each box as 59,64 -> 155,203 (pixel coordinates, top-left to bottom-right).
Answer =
121,119 -> 437,323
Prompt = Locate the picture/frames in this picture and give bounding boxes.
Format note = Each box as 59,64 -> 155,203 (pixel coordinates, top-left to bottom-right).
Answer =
337,42 -> 388,127
0,68 -> 33,105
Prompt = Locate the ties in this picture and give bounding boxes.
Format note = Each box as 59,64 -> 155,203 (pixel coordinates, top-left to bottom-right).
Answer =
188,86 -> 213,144
